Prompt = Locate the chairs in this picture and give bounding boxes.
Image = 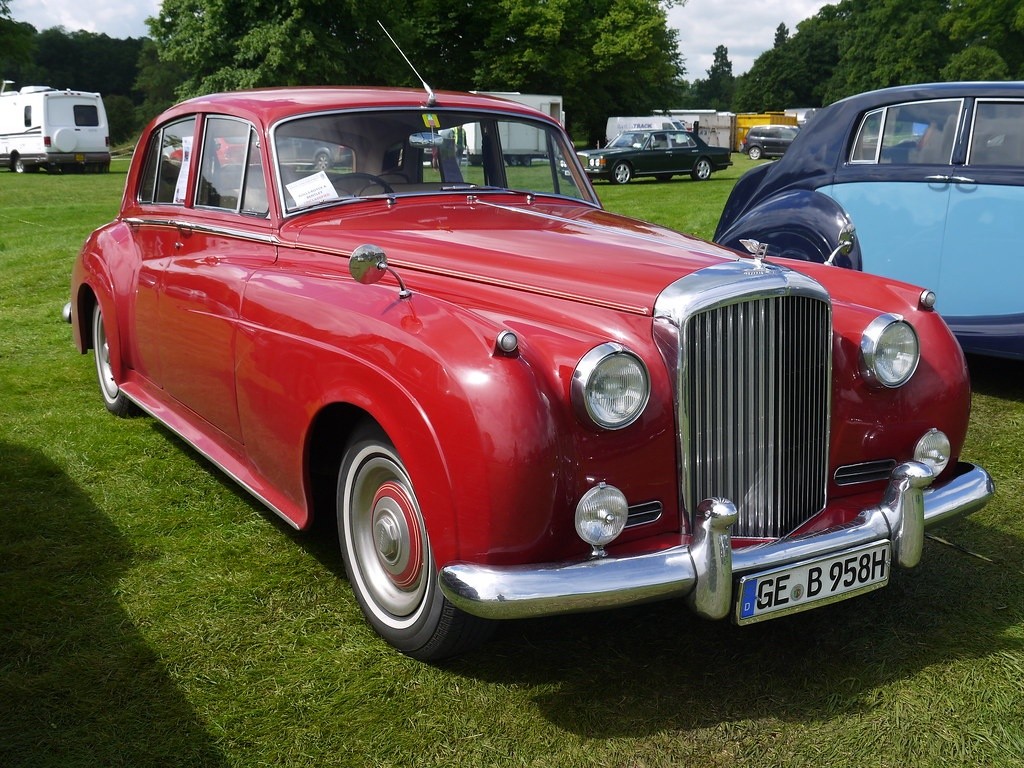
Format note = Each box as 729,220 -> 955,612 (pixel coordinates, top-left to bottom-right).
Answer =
143,164 -> 475,215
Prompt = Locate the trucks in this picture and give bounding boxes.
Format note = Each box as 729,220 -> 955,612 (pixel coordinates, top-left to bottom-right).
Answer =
438,91 -> 565,166
606,117 -> 688,147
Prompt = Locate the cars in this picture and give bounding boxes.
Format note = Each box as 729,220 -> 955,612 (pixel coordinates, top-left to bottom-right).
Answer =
557,129 -> 733,184
740,125 -> 798,160
69,91 -> 995,657
409,132 -> 443,162
162,135 -> 353,172
711,81 -> 1024,334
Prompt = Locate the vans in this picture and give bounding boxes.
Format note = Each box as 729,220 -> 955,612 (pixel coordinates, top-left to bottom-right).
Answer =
0,81 -> 112,174
